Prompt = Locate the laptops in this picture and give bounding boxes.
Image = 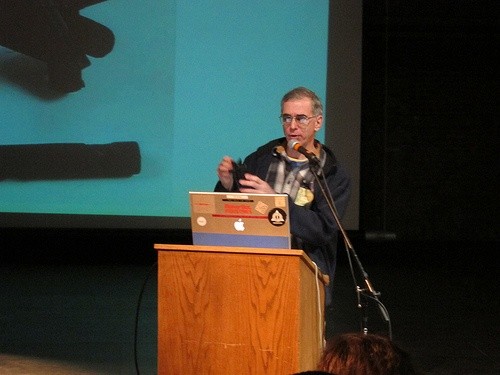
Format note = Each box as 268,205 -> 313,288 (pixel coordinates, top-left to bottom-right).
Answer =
189,192 -> 292,249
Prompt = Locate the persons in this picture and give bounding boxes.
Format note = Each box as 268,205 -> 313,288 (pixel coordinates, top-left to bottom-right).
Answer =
214,87 -> 354,338
315,333 -> 416,375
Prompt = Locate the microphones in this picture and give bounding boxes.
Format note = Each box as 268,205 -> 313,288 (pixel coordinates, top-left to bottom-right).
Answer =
287,138 -> 321,165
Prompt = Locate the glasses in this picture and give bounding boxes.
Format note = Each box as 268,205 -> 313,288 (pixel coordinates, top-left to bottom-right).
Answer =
278,113 -> 318,125
